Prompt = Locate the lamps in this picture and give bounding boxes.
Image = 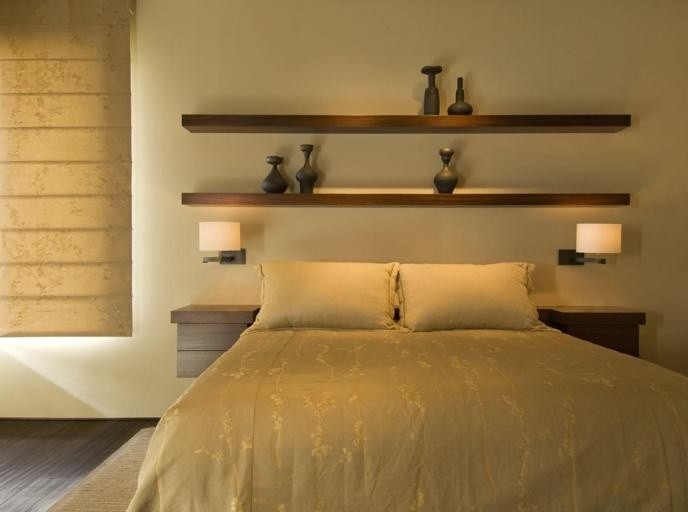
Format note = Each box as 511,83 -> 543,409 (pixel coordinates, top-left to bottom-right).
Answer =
197,221 -> 248,266
557,223 -> 623,265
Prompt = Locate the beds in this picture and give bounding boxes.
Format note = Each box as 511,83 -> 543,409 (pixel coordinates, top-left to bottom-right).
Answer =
127,260 -> 687,512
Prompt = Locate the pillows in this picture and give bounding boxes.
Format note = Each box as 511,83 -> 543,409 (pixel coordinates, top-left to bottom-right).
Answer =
392,260 -> 548,333
241,263 -> 401,331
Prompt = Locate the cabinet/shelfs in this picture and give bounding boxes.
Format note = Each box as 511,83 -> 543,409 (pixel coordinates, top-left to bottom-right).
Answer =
181,115 -> 632,204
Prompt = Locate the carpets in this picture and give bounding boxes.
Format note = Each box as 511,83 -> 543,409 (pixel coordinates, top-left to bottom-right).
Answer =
44,427 -> 152,511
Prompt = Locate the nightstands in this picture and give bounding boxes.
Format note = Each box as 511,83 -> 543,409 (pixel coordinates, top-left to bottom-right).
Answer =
171,303 -> 258,379
535,304 -> 646,357
